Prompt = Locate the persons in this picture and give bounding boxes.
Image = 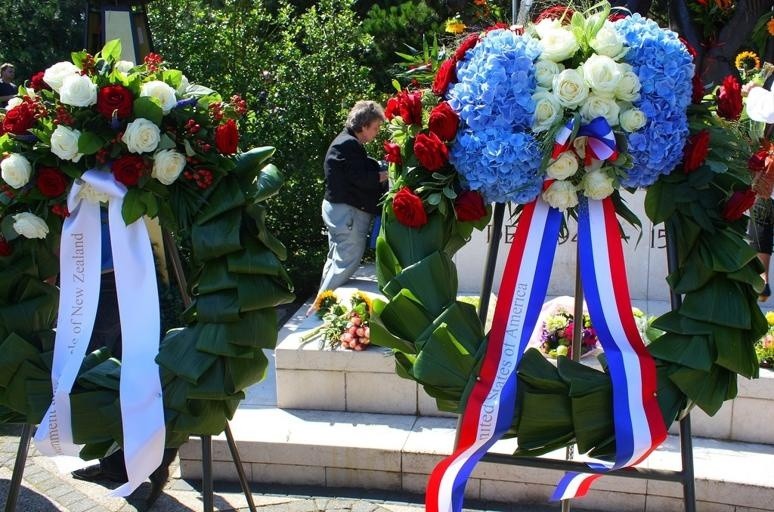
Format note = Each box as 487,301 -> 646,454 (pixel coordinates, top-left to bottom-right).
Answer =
744,156 -> 773,305
301,99 -> 394,319
